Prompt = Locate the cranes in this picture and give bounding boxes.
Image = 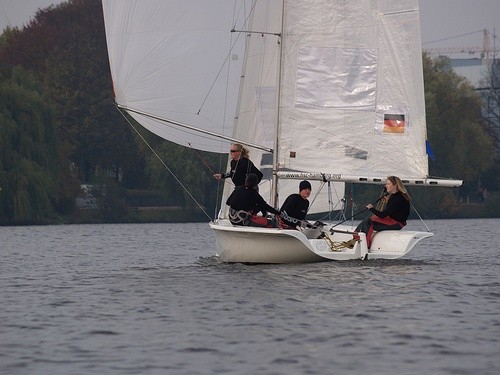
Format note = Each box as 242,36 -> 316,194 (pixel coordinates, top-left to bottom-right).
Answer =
421,28 -> 500,61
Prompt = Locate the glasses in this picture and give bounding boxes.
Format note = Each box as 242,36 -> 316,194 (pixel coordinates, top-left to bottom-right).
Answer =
231,149 -> 240,153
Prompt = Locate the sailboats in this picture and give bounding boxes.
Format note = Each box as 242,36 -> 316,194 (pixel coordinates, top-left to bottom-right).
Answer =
101,0 -> 463,265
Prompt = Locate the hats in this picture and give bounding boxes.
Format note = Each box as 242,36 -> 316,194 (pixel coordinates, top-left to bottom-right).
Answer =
300,180 -> 311,191
245,174 -> 258,186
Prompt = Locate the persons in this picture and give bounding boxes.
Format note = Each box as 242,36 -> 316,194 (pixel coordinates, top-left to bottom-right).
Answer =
212,143 -> 264,206
342,176 -> 411,251
228,174 -> 287,228
274,180 -> 311,231
